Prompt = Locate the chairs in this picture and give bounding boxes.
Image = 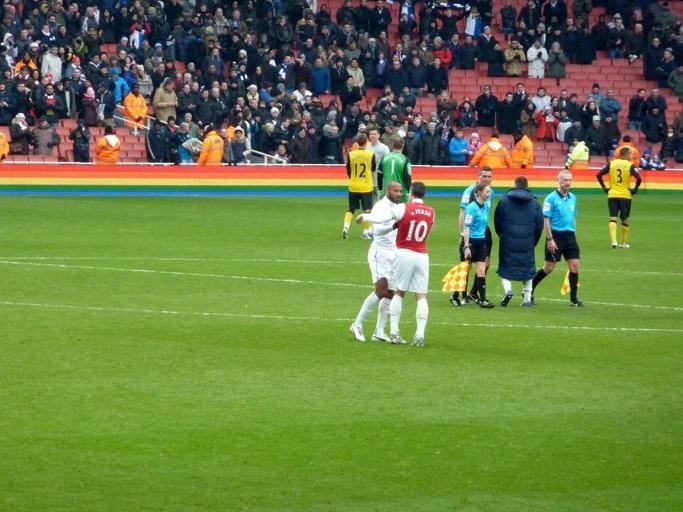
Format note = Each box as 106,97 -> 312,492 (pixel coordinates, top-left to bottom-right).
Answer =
0,0 -> 682,170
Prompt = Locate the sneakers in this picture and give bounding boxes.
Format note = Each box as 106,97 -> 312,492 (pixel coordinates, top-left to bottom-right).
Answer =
450,293 -> 494,308
520,291 -> 534,306
611,243 -> 617,248
569,298 -> 584,307
361,230 -> 372,239
342,229 -> 348,240
501,292 -> 513,306
350,322 -> 424,346
618,242 -> 630,248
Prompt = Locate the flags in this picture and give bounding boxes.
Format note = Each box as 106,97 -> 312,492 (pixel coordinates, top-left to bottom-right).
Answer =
442,261 -> 469,291
560,268 -> 582,296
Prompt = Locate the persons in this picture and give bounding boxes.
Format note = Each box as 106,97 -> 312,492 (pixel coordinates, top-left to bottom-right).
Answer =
450,165 -> 496,308
522,169 -> 585,308
1,1 -> 683,170
349,181 -> 436,347
494,176 -> 544,307
596,148 -> 642,248
341,127 -> 412,240
